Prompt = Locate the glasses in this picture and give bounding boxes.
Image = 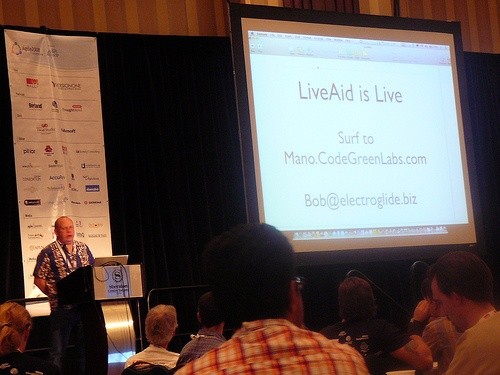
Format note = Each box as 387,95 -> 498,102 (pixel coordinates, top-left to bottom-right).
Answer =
289,275 -> 305,289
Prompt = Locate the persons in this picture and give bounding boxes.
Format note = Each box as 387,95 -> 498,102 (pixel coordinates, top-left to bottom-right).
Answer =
33,217 -> 95,364
0,221 -> 500,375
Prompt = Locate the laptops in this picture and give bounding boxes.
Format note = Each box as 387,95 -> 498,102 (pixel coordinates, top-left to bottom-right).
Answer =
94,255 -> 129,267
